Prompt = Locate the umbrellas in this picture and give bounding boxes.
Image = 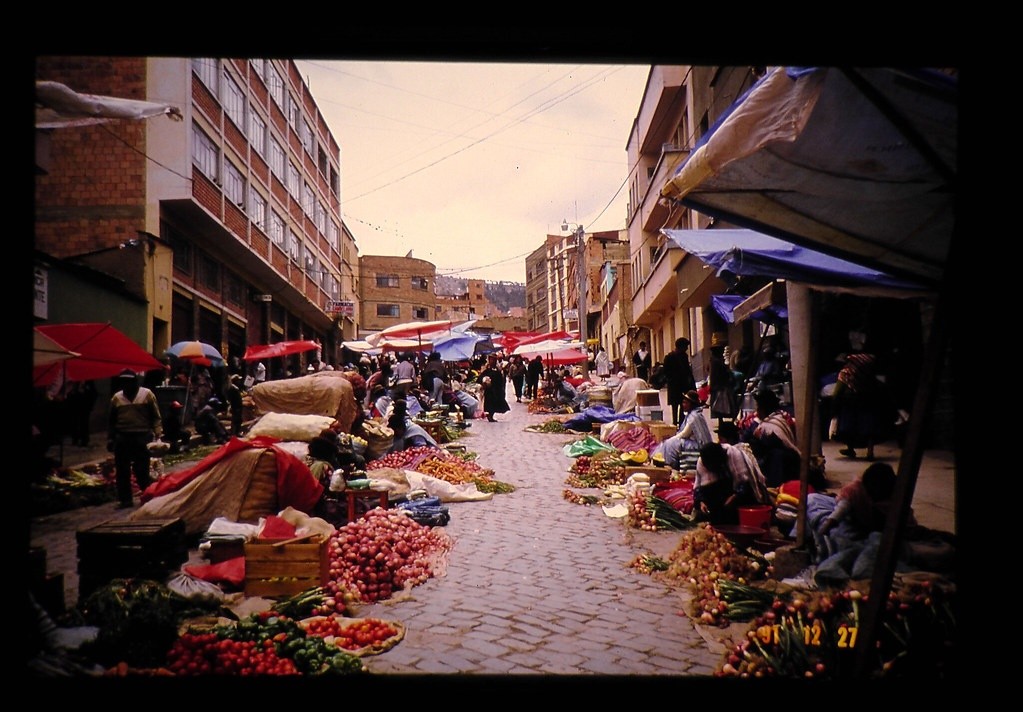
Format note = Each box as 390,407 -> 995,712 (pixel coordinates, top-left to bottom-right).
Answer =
32,327 -> 80,367
340,320 -> 599,366
31,319 -> 171,467
163,339 -> 229,425
242,338 -> 321,362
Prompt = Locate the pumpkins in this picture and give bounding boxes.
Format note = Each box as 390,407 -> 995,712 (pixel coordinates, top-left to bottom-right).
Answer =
620,447 -> 648,466
652,452 -> 666,468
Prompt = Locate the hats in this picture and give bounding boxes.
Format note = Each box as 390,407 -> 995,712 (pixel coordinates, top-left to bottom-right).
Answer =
676,337 -> 691,348
118,370 -> 137,378
209,397 -> 222,405
682,390 -> 703,405
231,374 -> 242,381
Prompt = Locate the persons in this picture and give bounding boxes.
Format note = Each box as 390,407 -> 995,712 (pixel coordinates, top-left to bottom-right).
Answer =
665,337 -> 694,429
695,390 -> 807,527
710,335 -> 888,461
229,345 -> 630,453
106,367 -> 163,510
805,463 -> 919,565
651,390 -> 711,466
632,341 -> 652,384
649,361 -> 665,390
145,356 -> 227,447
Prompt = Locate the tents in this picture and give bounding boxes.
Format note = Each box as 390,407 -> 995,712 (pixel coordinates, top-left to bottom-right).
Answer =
656,68 -> 959,319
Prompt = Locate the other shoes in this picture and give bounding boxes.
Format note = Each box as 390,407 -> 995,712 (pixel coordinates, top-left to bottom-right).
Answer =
861,455 -> 875,462
487,414 -> 495,422
224,436 -> 230,441
517,399 -> 519,401
218,440 -> 227,444
519,400 -> 521,403
840,448 -> 856,458
114,501 -> 134,510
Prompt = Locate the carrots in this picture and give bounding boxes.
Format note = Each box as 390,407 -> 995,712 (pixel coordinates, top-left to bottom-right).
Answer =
104,662 -> 177,676
415,458 -> 489,485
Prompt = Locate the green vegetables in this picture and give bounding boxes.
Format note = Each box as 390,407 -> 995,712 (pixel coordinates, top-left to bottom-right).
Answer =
537,420 -> 569,433
55,574 -> 218,661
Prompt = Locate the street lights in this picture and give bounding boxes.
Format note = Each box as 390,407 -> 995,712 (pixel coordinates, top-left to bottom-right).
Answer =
561,219 -> 588,379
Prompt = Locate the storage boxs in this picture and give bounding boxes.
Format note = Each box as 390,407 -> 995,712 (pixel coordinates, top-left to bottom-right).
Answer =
635,390 -> 677,443
77,517 -> 190,600
243,534 -> 329,598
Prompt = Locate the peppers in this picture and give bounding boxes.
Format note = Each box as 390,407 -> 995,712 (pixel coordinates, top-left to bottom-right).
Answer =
188,612 -> 371,674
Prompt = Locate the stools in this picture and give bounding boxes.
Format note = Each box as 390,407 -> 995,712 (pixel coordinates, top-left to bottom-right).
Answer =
416,422 -> 442,443
345,489 -> 389,522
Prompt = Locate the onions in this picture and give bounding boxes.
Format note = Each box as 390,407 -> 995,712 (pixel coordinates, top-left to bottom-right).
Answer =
369,446 -> 482,474
97,469 -> 140,495
311,507 -> 439,617
712,580 -> 932,678
624,491 -> 657,531
663,524 -> 776,623
563,454 -> 625,507
632,553 -> 650,575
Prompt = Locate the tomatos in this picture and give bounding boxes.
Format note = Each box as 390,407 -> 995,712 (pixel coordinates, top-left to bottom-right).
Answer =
163,632 -> 300,674
259,609 -> 397,650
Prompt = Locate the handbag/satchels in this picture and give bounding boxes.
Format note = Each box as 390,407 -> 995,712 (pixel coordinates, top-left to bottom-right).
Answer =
244,375 -> 255,388
711,389 -> 730,414
